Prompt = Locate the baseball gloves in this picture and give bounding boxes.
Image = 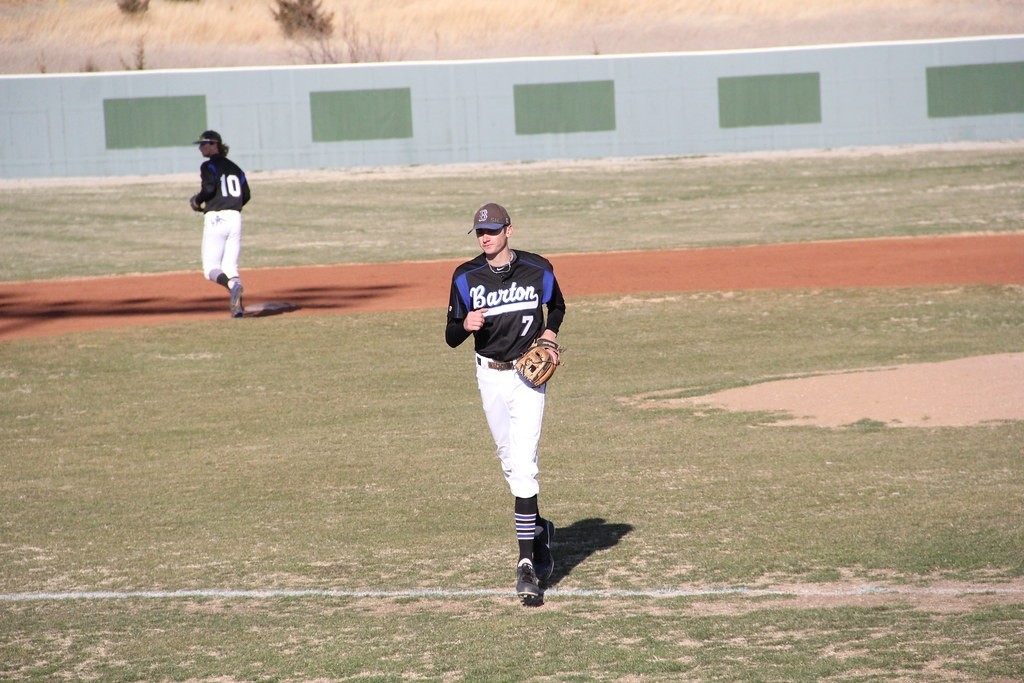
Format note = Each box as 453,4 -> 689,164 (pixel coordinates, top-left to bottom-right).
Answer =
189,194 -> 204,212
513,338 -> 567,389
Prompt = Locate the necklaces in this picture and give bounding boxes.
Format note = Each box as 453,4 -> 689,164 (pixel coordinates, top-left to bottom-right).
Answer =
485,252 -> 511,273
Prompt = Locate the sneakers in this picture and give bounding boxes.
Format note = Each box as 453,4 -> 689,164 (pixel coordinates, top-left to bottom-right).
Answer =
516,563 -> 539,605
531,518 -> 555,581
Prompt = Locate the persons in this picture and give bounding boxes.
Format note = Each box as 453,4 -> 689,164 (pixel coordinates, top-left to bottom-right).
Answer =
445,203 -> 566,597
190,130 -> 251,318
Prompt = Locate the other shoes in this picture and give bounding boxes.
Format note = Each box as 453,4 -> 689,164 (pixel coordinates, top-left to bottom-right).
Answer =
232,307 -> 243,317
230,282 -> 243,313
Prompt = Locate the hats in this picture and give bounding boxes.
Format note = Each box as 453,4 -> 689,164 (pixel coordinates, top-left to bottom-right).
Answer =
192,130 -> 222,144
468,203 -> 510,235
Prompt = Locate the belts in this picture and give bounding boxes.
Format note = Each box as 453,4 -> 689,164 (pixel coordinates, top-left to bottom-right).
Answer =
477,357 -> 514,372
